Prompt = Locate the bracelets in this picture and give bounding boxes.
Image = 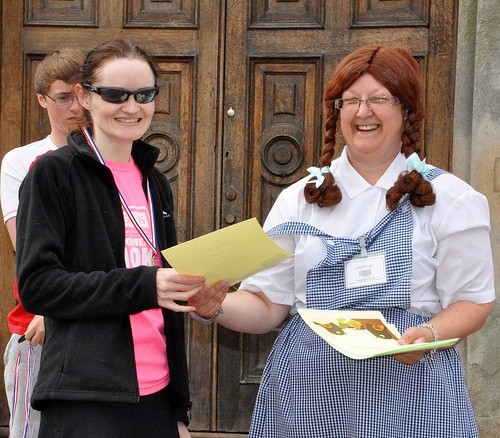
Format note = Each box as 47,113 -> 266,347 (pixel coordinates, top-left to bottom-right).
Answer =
417,323 -> 439,354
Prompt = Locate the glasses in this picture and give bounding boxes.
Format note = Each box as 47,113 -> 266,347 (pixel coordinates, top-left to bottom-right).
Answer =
80,81 -> 160,104
334,97 -> 401,110
46,94 -> 78,106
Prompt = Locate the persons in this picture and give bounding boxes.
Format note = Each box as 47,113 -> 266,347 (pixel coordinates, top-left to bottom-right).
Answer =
187,45 -> 496,438
15,38 -> 207,438
0,48 -> 87,438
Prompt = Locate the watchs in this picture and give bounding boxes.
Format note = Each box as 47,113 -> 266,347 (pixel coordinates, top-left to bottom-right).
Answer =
190,308 -> 220,324
